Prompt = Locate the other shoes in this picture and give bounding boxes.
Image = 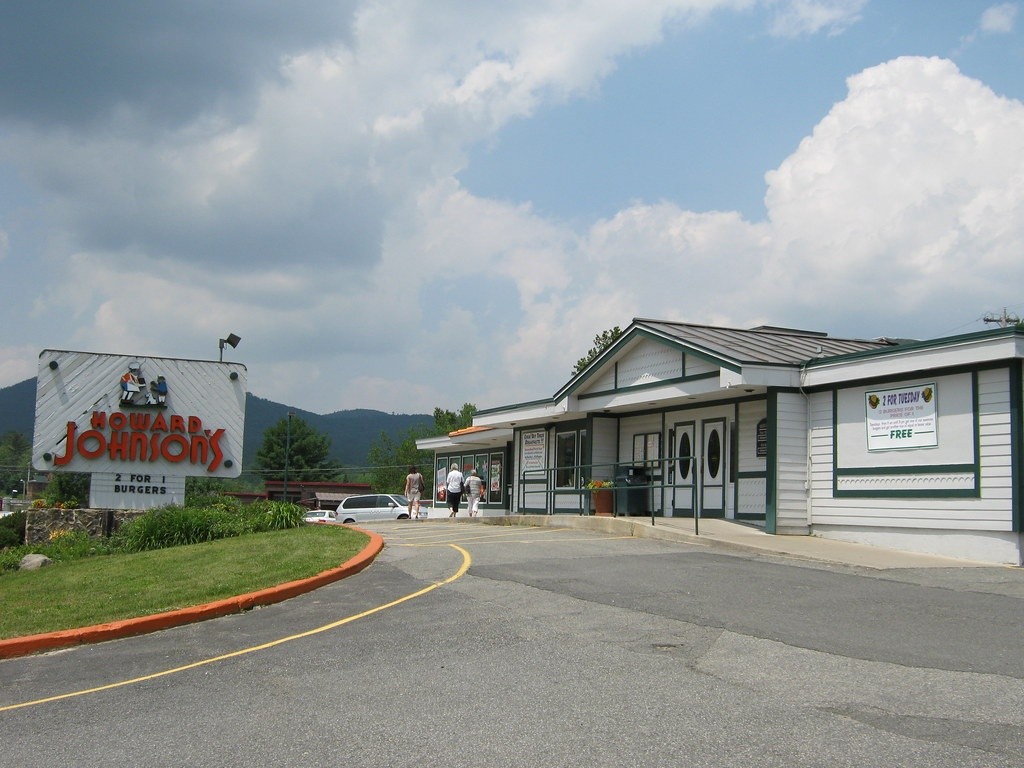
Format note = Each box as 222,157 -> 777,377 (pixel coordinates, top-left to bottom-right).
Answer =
470,512 -> 472,517
415,516 -> 418,519
453,513 -> 456,517
449,511 -> 454,518
408,516 -> 411,519
474,513 -> 476,517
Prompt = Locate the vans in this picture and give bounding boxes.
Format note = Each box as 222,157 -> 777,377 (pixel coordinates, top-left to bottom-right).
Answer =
335,494 -> 428,524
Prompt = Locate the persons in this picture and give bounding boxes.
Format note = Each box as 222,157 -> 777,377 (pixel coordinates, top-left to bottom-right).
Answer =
447,463 -> 465,517
463,469 -> 482,517
405,466 -> 426,519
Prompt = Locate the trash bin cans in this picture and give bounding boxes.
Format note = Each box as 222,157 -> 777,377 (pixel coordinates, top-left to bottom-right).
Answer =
12,490 -> 18,498
615,466 -> 648,516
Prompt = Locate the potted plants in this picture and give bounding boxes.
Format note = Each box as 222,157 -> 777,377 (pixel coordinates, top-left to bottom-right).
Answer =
587,479 -> 616,516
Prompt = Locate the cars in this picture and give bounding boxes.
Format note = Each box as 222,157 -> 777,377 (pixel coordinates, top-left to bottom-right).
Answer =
301,510 -> 336,524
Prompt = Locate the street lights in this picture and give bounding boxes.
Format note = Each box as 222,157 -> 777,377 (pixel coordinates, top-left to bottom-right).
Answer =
20,479 -> 25,500
283,412 -> 296,500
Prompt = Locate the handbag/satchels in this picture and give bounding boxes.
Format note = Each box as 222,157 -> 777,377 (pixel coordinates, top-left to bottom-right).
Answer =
418,474 -> 425,493
465,477 -> 471,494
460,476 -> 465,495
